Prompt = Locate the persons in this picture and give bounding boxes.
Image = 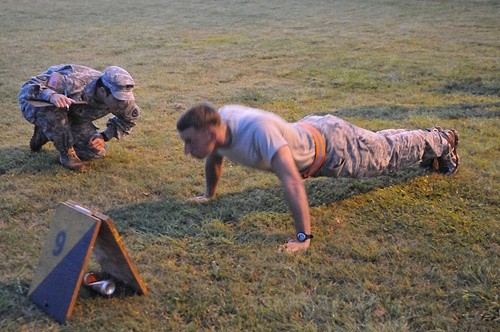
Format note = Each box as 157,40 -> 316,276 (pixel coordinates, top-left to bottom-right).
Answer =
18,64 -> 140,170
177,101 -> 460,253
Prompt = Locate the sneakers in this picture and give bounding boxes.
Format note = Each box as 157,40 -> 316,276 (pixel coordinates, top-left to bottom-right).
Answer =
58,148 -> 86,172
439,128 -> 460,176
426,125 -> 442,171
29,124 -> 49,152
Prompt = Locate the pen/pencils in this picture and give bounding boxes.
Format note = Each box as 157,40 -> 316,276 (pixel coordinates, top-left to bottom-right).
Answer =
63,88 -> 70,112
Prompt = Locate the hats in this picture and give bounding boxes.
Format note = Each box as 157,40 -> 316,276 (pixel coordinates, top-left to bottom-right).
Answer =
101,65 -> 136,100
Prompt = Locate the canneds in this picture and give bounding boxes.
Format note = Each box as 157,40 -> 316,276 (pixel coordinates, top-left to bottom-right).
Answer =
83,272 -> 116,295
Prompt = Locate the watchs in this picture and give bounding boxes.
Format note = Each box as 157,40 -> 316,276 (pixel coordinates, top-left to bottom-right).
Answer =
296,232 -> 313,242
